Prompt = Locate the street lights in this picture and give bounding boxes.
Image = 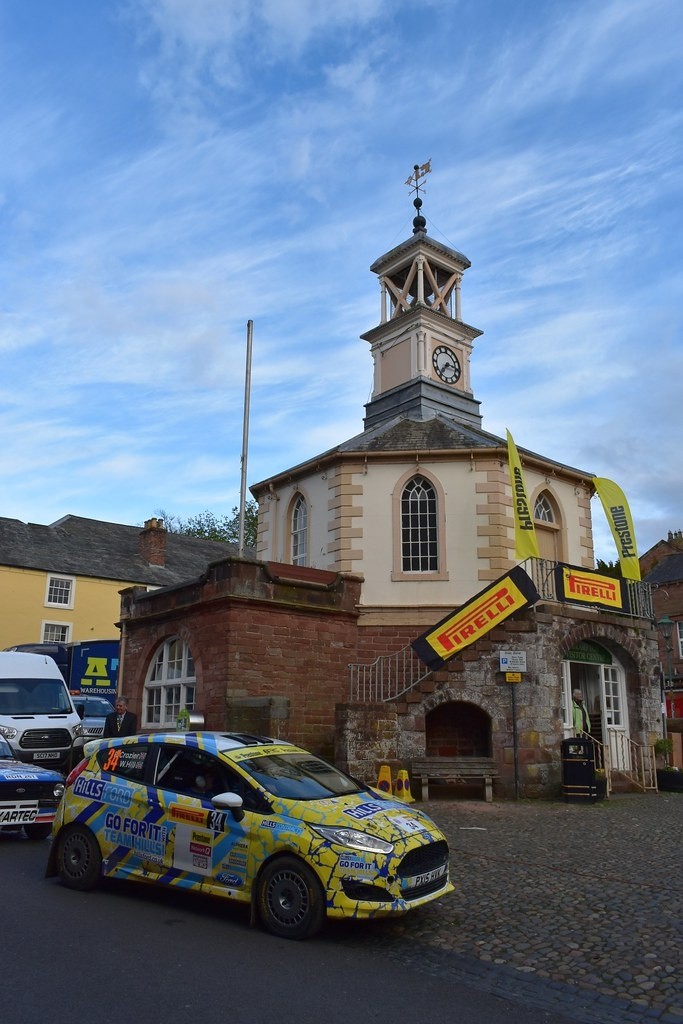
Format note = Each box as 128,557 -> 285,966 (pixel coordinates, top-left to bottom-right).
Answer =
656,612 -> 675,722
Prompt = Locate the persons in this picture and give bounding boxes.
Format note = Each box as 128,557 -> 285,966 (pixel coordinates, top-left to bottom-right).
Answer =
103,695 -> 138,739
572,688 -> 592,752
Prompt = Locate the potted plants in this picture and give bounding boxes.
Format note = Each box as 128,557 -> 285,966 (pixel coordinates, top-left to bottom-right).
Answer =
654,738 -> 683,793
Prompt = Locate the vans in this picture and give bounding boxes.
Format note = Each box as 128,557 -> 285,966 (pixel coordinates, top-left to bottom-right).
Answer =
0,651 -> 87,775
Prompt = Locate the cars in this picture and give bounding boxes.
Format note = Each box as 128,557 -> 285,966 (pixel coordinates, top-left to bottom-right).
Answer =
0,732 -> 65,842
44,733 -> 455,938
68,689 -> 114,735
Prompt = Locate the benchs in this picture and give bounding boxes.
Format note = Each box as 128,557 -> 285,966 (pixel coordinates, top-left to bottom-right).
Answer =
410,756 -> 501,803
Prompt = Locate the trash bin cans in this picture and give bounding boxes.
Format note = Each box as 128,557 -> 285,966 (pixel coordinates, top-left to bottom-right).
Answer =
561,736 -> 597,804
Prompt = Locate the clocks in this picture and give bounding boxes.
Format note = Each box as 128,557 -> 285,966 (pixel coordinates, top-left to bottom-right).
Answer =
431,344 -> 462,384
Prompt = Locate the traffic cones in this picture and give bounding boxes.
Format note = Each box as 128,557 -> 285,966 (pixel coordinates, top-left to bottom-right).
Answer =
376,766 -> 393,796
394,769 -> 415,804
